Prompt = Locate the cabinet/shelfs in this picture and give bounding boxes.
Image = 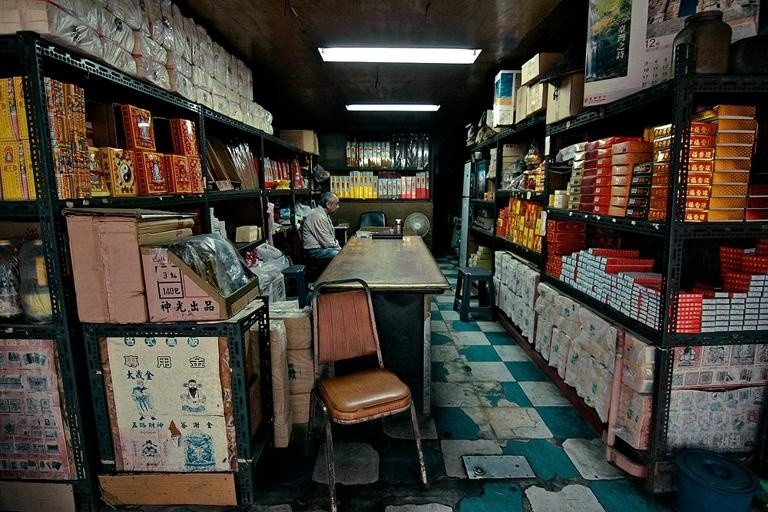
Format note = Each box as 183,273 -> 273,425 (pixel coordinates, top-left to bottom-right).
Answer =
1,29 -> 323,512
464,41 -> 768,505
312,125 -> 434,204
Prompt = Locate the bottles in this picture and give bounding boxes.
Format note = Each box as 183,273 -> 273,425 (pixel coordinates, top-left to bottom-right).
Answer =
392,218 -> 405,233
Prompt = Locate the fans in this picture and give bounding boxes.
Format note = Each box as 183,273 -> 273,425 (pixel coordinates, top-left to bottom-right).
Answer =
404,212 -> 431,238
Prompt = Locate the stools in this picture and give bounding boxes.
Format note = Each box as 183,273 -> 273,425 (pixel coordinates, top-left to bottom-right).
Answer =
453,267 -> 499,322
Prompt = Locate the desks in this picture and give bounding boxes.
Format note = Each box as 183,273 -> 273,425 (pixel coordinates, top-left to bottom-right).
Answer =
313,233 -> 452,420
333,223 -> 352,244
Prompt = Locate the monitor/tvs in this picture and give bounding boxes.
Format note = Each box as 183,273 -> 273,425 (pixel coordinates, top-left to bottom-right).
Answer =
476,160 -> 488,199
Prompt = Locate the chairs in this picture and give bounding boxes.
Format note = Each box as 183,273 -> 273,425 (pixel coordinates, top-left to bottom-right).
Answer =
310,277 -> 429,512
352,210 -> 387,236
291,230 -> 333,267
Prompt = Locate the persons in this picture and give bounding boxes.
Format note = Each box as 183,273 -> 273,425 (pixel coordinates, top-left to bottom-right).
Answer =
131,378 -> 157,420
180,378 -> 208,412
300,191 -> 343,259
142,439 -> 158,466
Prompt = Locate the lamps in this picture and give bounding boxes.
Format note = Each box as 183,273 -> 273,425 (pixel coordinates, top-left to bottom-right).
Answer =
316,41 -> 483,65
345,98 -> 441,112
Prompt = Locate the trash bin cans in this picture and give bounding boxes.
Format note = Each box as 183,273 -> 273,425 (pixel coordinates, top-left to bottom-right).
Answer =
676,448 -> 761,512
